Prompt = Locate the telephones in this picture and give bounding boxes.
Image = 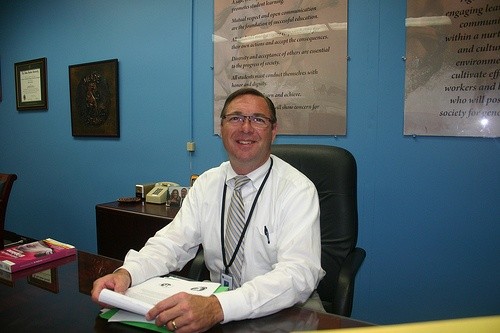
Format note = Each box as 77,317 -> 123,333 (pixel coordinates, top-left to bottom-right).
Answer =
146,181 -> 180,204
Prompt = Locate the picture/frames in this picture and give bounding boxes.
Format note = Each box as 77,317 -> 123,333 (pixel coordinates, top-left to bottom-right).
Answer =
68,59 -> 121,139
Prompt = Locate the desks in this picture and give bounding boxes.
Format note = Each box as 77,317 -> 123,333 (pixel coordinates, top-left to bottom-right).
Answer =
0,229 -> 379,333
95,198 -> 210,281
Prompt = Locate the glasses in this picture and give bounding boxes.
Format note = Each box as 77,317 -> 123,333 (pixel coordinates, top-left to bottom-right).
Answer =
219,114 -> 274,127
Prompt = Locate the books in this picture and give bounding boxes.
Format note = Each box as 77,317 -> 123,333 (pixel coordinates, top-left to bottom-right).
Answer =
0,238 -> 76,273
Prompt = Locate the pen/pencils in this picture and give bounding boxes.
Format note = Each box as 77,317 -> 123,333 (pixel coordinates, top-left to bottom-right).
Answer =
263,226 -> 270,244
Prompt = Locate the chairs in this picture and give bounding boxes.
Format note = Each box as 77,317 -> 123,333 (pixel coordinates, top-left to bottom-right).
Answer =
0,173 -> 18,230
188,144 -> 366,319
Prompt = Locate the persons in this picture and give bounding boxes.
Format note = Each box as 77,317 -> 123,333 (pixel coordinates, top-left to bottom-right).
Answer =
91,88 -> 326,333
168,188 -> 187,207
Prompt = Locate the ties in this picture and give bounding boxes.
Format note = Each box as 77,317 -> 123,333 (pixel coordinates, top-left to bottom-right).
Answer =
225,175 -> 251,290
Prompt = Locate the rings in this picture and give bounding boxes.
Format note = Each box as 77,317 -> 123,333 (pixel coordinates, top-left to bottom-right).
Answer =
172,321 -> 177,329
